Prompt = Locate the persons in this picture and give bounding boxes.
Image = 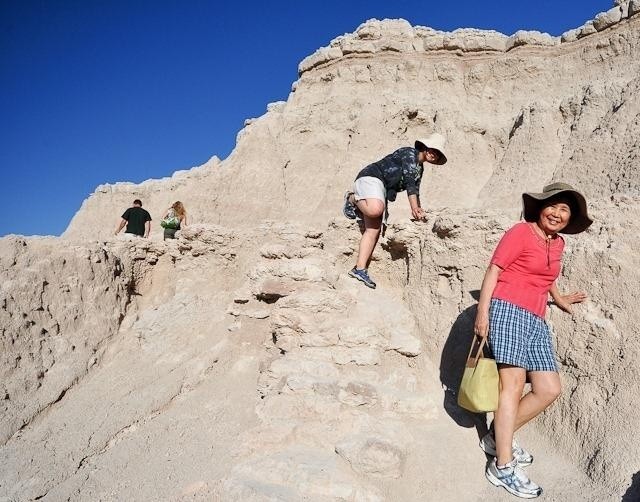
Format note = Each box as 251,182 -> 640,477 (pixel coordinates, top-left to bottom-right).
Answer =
343,132 -> 448,290
162,201 -> 189,242
115,199 -> 151,239
474,181 -> 594,500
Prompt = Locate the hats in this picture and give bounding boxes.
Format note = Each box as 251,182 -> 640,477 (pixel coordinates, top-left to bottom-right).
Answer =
522,182 -> 593,235
414,132 -> 449,165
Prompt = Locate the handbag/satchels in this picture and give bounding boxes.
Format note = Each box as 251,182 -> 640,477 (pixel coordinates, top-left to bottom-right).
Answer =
457,334 -> 501,412
160,208 -> 182,228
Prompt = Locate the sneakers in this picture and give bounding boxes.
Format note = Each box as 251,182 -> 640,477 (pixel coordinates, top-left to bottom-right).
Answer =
479,428 -> 534,468
343,190 -> 357,219
347,265 -> 376,288
485,456 -> 544,498
